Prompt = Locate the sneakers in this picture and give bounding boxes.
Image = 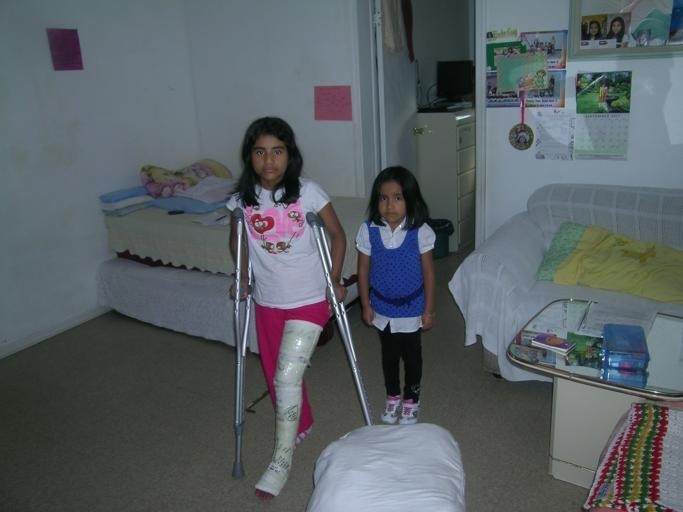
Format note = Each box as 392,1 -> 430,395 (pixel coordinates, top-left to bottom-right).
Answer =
381,395 -> 403,424
398,399 -> 422,426
296,425 -> 313,446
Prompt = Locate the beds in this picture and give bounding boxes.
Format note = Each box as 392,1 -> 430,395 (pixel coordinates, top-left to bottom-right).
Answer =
98,157 -> 372,357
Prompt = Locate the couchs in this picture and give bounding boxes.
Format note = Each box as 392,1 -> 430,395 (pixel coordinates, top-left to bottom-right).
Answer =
468,181 -> 682,379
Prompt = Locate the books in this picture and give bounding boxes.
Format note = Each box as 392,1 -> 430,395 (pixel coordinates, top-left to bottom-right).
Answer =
531,333 -> 576,352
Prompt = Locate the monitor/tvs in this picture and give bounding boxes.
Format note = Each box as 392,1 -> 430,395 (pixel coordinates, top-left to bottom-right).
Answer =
436,60 -> 474,102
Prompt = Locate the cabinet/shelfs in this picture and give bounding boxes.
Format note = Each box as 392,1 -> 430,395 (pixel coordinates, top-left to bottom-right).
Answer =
417,106 -> 476,253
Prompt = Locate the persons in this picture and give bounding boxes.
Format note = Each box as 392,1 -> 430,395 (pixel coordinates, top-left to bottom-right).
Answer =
585,19 -> 603,42
596,80 -> 610,104
225,114 -> 348,501
353,164 -> 437,427
604,16 -> 631,45
635,30 -> 649,47
515,130 -> 528,146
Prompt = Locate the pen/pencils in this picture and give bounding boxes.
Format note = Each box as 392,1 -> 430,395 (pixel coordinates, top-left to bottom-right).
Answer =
216,215 -> 227,221
577,300 -> 593,332
